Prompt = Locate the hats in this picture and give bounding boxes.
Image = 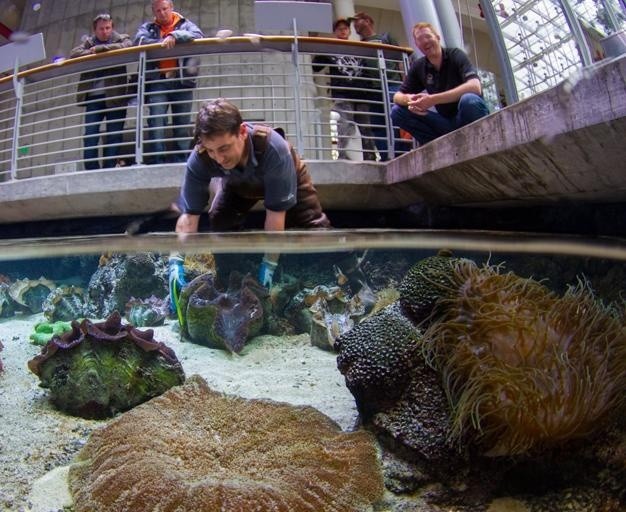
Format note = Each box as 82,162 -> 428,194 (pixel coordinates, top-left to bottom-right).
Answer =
346,10 -> 374,25
332,16 -> 350,32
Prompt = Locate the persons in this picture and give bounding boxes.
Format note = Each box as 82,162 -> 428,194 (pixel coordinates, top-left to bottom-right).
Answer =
311,15 -> 379,161
389,21 -> 490,145
348,11 -> 412,161
168,96 -> 376,316
69,13 -> 133,170
134,0 -> 206,164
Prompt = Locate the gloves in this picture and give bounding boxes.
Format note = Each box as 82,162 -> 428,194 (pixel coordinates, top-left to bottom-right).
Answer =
167,256 -> 189,314
258,257 -> 279,297
